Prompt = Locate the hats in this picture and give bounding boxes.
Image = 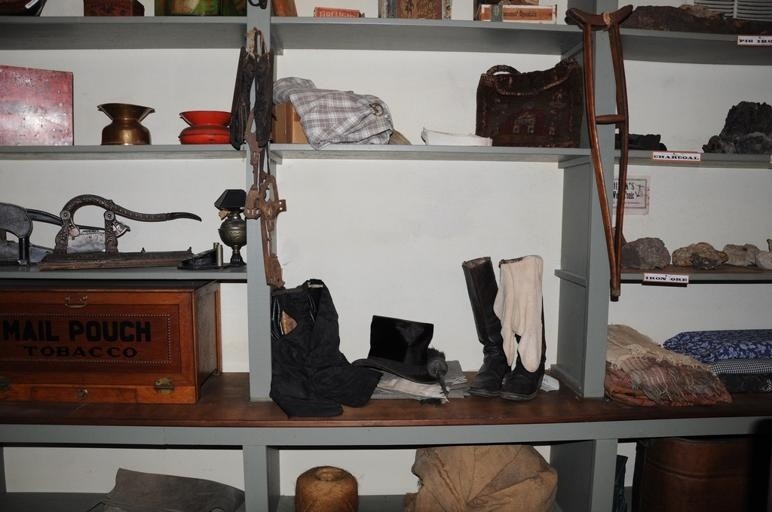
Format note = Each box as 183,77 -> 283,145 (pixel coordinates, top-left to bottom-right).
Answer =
353,315 -> 437,385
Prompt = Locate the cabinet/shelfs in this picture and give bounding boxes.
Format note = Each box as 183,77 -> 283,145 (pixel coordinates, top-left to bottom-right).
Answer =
0,0 -> 772,512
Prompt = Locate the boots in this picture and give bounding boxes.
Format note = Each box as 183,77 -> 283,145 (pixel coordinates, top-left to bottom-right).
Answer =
462,255 -> 547,401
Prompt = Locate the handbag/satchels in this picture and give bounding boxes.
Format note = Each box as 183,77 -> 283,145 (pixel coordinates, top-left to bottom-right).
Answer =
269,279 -> 381,417
475,58 -> 583,147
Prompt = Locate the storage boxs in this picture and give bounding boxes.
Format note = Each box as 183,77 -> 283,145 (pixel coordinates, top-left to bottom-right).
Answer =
1,278 -> 218,404
